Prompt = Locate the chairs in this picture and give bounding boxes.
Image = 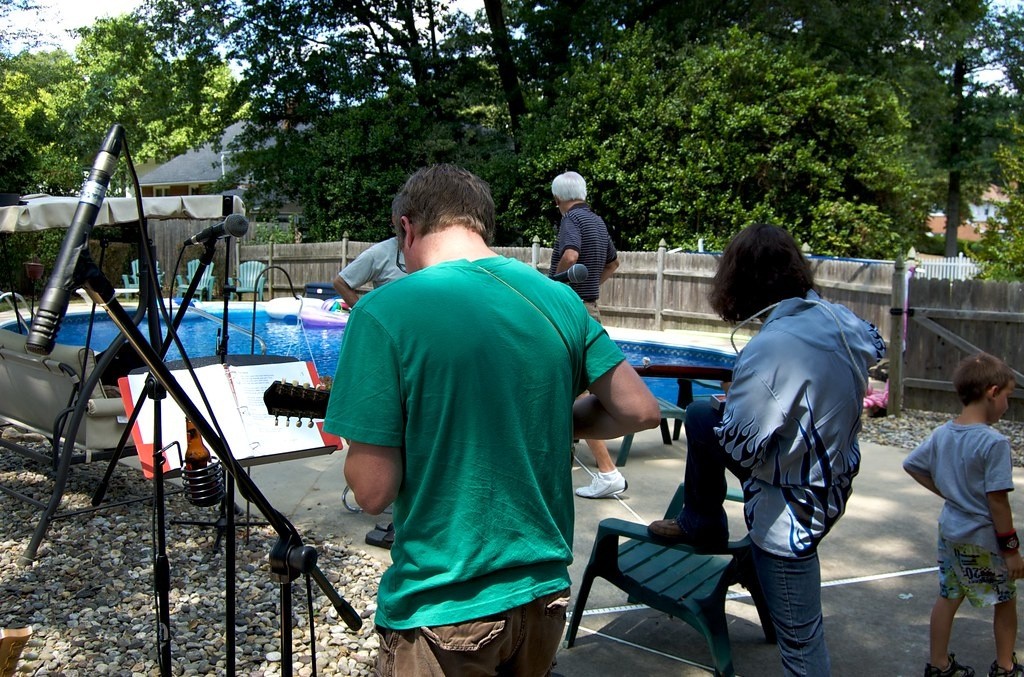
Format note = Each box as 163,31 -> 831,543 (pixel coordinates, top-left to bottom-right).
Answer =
177,259 -> 216,302
122,258 -> 165,305
571,370 -> 695,466
564,480 -> 786,677
228,260 -> 268,301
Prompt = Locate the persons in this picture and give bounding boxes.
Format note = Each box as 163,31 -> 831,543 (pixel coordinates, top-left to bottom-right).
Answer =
323,163 -> 660,677
332,195 -> 409,306
548,171 -> 628,499
647,222 -> 888,677
902,351 -> 1024,677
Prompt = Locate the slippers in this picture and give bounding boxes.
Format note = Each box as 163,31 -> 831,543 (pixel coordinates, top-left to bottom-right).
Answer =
365,522 -> 400,550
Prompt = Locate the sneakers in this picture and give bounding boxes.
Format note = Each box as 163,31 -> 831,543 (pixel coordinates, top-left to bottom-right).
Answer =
576,468 -> 630,499
923,652 -> 976,677
987,652 -> 1024,677
648,520 -> 731,554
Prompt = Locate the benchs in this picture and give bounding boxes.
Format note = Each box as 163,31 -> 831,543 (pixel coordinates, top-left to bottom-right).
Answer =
0,329 -> 246,566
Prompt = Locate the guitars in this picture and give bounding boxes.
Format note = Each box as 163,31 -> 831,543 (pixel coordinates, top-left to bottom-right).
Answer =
260,375 -> 330,429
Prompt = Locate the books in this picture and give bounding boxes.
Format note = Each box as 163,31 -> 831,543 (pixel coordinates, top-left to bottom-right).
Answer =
117,360 -> 343,479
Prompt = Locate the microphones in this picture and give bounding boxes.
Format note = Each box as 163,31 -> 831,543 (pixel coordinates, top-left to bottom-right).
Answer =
25,123 -> 124,356
548,264 -> 588,283
184,214 -> 249,246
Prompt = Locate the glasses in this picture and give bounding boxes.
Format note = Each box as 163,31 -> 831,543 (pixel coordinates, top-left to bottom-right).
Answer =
395,244 -> 408,273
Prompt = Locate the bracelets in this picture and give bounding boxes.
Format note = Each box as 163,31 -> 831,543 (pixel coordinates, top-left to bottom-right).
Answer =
995,528 -> 1020,553
